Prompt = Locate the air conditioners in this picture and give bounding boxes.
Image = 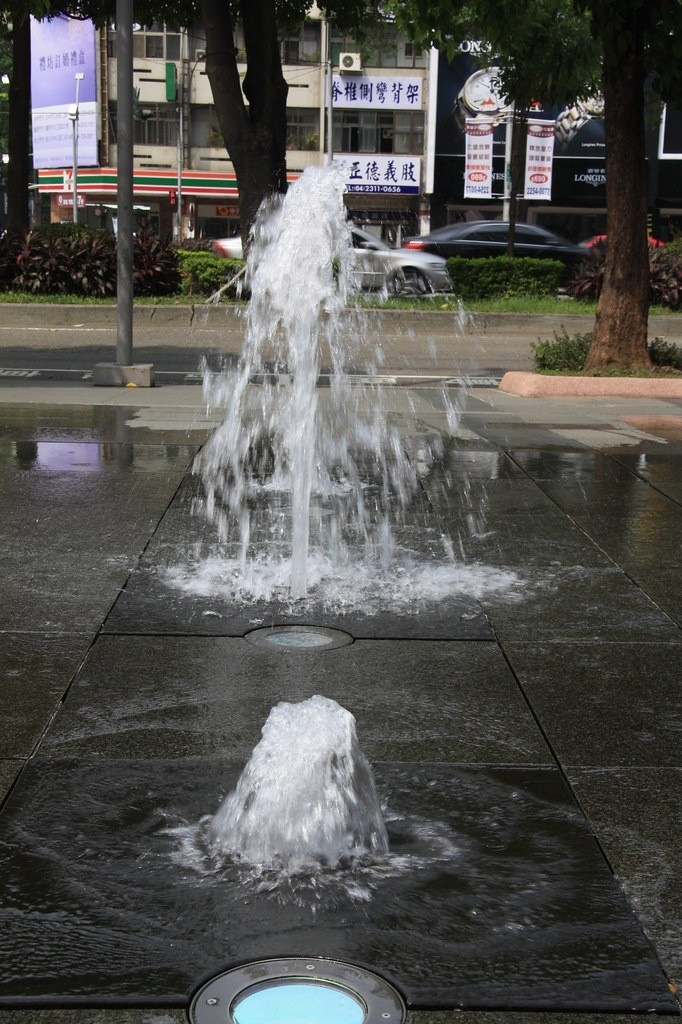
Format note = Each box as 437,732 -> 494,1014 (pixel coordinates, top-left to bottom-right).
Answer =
338,52 -> 363,71
194,48 -> 207,62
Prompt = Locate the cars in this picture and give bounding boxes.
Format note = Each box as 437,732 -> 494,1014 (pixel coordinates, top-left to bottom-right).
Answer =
211,221 -> 453,298
401,220 -> 601,294
578,234 -> 668,254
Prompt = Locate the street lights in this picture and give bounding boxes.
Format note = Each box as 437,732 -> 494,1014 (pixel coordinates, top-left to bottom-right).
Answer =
67,72 -> 86,225
